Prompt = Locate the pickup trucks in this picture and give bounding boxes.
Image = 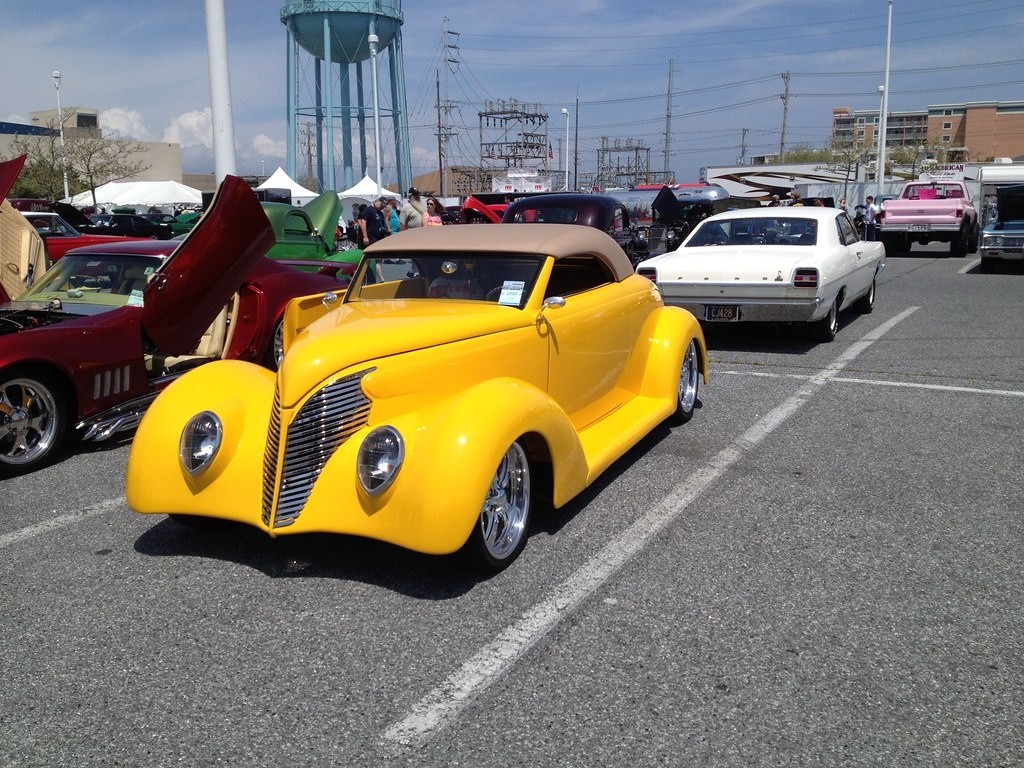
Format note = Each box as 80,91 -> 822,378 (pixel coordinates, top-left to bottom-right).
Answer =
874,195 -> 899,234
880,180 -> 980,259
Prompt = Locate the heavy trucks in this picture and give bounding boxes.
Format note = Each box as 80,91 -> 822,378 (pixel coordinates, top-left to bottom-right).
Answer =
698,164 -> 876,205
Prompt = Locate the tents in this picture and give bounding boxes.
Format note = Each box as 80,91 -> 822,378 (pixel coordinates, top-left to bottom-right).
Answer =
252,166 -> 401,233
59,180 -> 202,217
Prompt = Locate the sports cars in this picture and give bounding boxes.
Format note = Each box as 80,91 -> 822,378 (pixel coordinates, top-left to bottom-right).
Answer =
0,153 -> 359,474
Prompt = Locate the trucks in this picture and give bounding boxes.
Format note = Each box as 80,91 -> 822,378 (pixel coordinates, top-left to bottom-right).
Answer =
979,164 -> 1024,264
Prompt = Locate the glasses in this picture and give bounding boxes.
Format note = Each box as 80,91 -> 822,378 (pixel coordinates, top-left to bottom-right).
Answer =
427,203 -> 435,205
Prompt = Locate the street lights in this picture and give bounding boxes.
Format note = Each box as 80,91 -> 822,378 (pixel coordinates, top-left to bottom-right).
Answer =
52,71 -> 70,199
563,108 -> 570,193
369,35 -> 382,200
876,86 -> 885,200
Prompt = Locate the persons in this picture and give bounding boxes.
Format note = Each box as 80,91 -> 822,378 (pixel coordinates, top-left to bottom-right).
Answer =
356,187 -> 446,283
767,193 -> 878,241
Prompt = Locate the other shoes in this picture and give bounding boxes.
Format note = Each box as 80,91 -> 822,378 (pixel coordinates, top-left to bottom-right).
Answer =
407,270 -> 421,279
395,259 -> 406,264
383,259 -> 395,264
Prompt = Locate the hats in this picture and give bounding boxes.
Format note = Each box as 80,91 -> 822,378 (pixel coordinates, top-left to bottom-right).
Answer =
380,197 -> 388,205
409,187 -> 421,195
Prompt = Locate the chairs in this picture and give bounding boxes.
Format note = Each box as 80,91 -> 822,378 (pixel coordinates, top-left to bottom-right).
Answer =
141,304 -> 227,372
918,188 -> 937,199
115,259 -> 158,294
796,234 -> 816,244
728,234 -> 752,245
427,269 -> 483,300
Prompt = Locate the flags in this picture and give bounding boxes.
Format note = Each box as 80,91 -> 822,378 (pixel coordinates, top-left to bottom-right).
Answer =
548,140 -> 553,160
489,151 -> 498,160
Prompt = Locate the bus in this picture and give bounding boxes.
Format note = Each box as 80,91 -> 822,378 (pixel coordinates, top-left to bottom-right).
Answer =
604,186 -> 731,240
461,193 -> 545,224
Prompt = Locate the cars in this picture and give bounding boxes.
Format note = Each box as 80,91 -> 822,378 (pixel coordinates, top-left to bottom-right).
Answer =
634,205 -> 887,344
127,225 -> 711,570
2,191 -> 508,285
502,193 -> 652,272
